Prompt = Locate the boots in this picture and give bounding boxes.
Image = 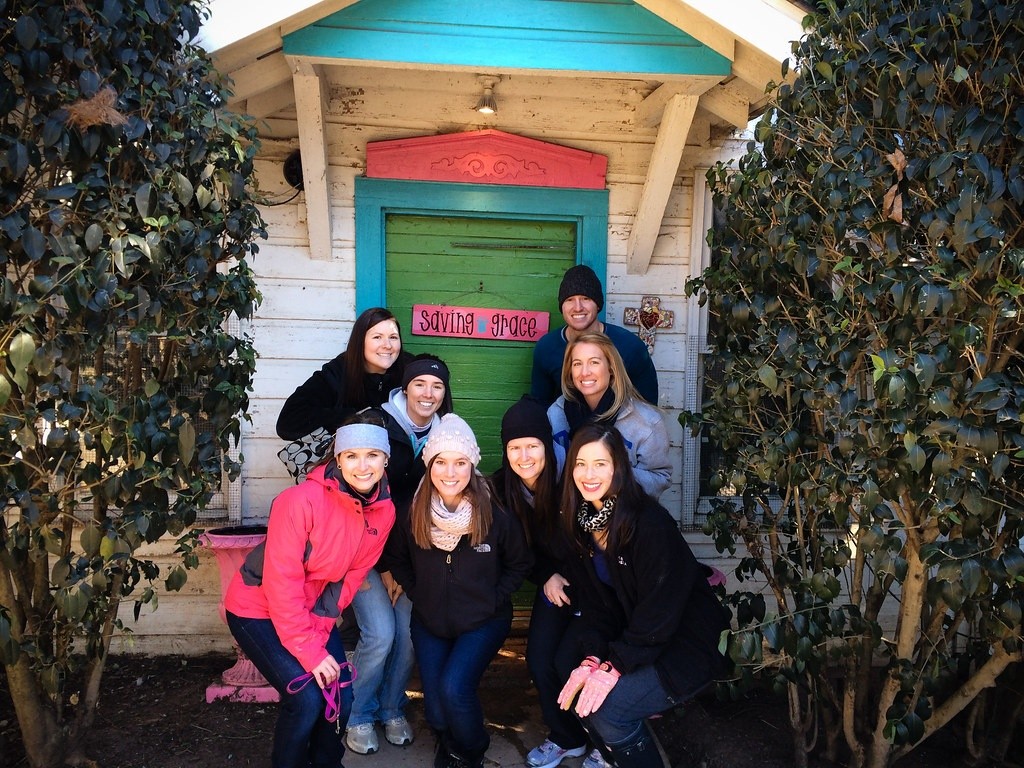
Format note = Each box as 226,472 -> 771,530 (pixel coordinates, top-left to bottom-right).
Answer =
575,710 -> 663,768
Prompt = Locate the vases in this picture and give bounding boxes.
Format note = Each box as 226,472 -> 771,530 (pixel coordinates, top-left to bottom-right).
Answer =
199,523 -> 282,704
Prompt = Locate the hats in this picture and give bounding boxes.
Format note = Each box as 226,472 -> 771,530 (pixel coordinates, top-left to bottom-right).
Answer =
420,412 -> 482,469
557,264 -> 605,315
500,397 -> 553,453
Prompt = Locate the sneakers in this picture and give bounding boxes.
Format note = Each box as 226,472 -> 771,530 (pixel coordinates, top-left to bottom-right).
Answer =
581,746 -> 616,768
526,740 -> 587,768
346,722 -> 379,755
382,715 -> 414,745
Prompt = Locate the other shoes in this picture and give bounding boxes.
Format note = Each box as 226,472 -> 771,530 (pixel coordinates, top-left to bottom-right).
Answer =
433,736 -> 484,767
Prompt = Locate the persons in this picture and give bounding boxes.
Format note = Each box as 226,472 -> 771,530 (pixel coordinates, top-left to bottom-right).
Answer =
345,354 -> 451,753
493,402 -> 587,768
391,413 -> 514,768
224,413 -> 396,768
275,308 -> 409,444
547,330 -> 673,499
532,264 -> 659,408
557,424 -> 734,768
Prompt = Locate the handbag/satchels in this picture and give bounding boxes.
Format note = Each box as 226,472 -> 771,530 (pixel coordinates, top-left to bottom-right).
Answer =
277,427 -> 336,485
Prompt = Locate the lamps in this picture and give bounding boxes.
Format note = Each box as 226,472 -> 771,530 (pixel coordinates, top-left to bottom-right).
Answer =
476,75 -> 501,114
